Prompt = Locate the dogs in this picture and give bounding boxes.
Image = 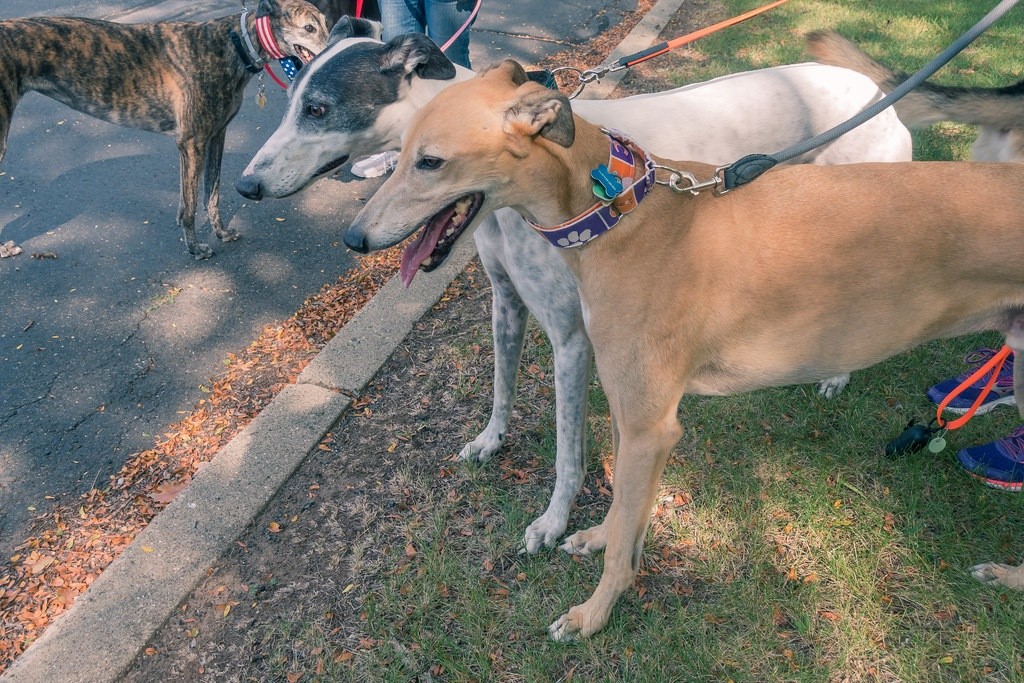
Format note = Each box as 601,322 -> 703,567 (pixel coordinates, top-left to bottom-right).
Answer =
235,16 -> 913,554
0,0 -> 330,261
344,60 -> 1024,643
800,27 -> 1023,165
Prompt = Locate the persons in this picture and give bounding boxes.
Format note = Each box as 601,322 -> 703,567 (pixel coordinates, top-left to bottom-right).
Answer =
925,344 -> 1024,492
351,0 -> 478,179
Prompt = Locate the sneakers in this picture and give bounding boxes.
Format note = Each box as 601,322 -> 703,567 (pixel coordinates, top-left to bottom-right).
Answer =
926,347 -> 1017,416
955,424 -> 1024,491
351,150 -> 401,178
